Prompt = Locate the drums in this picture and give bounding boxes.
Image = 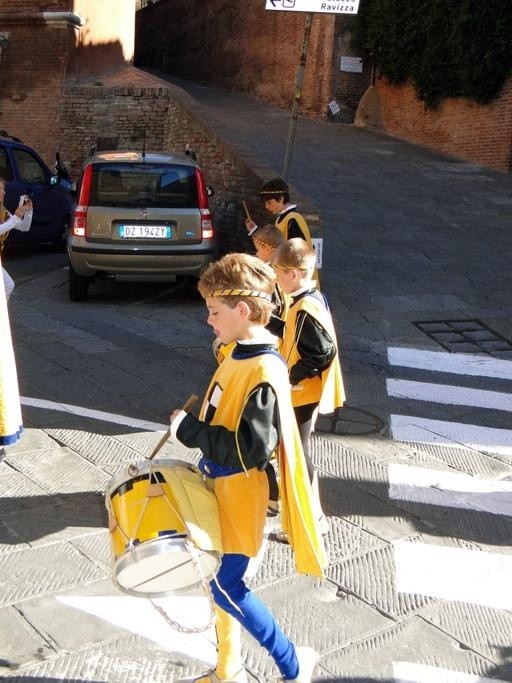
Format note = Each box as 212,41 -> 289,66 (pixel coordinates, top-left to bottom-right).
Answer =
212,337 -> 229,363
105,459 -> 223,598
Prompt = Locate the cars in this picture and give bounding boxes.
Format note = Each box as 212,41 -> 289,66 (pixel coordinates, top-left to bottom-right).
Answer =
68,143 -> 215,302
1,131 -> 75,257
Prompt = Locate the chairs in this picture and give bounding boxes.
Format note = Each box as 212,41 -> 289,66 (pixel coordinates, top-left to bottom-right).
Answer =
98,170 -> 132,201
155,170 -> 186,201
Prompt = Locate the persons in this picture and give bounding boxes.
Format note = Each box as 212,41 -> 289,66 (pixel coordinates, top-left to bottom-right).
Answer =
245,175 -> 323,293
263,235 -> 346,541
168,250 -> 319,682
0,265 -> 25,464
0,176 -> 35,303
250,224 -> 285,265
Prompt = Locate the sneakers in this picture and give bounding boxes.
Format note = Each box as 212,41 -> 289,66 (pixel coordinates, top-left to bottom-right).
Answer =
174,662 -> 250,682
272,645 -> 318,682
275,514 -> 331,542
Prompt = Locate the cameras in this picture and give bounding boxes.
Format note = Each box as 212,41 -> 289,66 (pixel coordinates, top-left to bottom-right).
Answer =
19,195 -> 29,207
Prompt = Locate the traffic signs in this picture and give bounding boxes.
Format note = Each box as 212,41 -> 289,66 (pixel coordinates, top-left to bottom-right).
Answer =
266,0 -> 359,15
